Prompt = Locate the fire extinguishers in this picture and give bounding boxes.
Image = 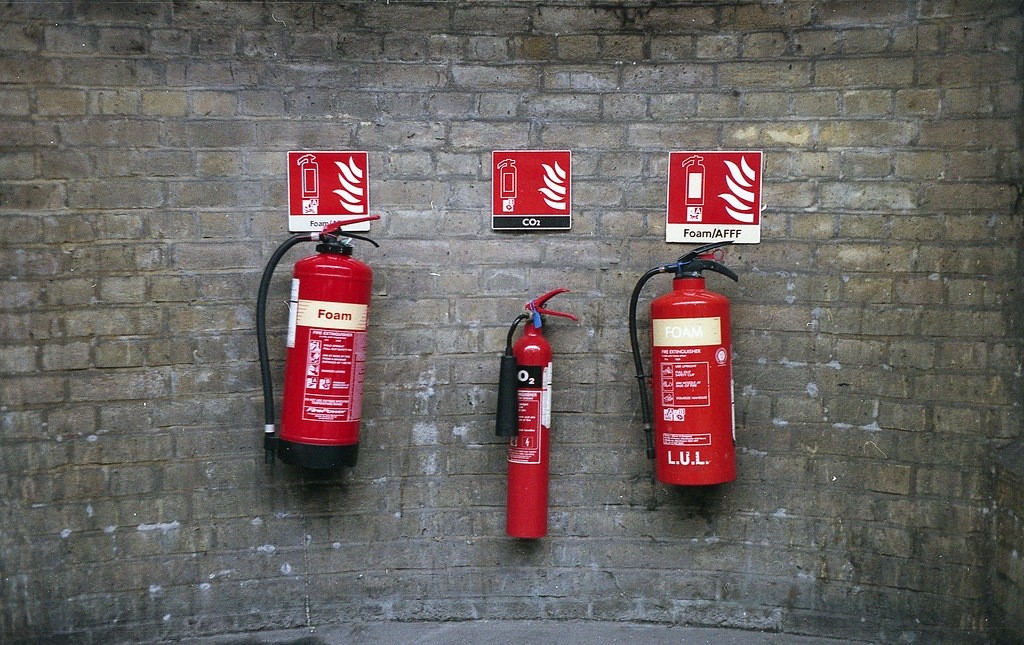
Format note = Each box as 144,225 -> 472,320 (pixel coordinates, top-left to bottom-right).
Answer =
629,240 -> 739,486
494,287 -> 578,539
256,214 -> 381,471
